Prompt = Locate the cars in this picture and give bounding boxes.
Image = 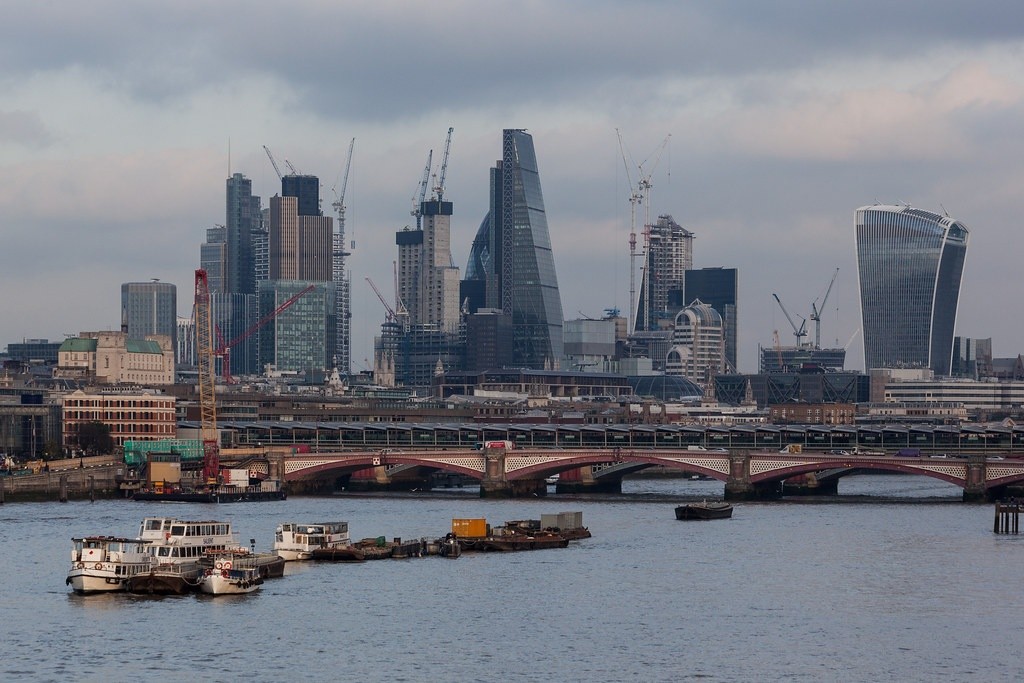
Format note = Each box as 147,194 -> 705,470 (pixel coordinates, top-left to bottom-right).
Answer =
710,448 -> 729,452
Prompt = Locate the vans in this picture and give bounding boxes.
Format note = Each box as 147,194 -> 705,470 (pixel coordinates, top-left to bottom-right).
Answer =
381,449 -> 392,452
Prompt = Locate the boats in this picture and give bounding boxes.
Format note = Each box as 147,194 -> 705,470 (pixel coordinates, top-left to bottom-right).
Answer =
198,549 -> 265,595
68,537 -> 155,593
133,517 -> 236,567
674,501 -> 733,521
442,542 -> 463,559
272,519 -> 352,562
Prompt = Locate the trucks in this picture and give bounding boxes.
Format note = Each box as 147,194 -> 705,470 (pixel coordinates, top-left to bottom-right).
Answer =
894,449 -> 920,456
780,445 -> 803,454
822,450 -> 835,455
688,445 -> 707,451
851,447 -> 885,456
474,440 -> 515,451
835,450 -> 850,456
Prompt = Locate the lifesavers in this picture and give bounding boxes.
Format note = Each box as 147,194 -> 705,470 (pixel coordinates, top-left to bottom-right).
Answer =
94,563 -> 102,571
77,563 -> 84,569
215,562 -> 223,569
224,562 -> 232,569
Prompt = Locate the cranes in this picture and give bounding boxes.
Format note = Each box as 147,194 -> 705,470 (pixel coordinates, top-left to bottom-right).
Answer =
808,266 -> 840,348
213,284 -> 316,382
331,136 -> 356,276
434,126 -> 455,200
263,145 -> 297,182
772,293 -> 810,347
408,148 -> 434,230
614,126 -> 676,337
365,276 -> 400,326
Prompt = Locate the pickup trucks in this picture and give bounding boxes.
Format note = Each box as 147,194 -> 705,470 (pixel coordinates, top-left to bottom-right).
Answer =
987,456 -> 1004,460
